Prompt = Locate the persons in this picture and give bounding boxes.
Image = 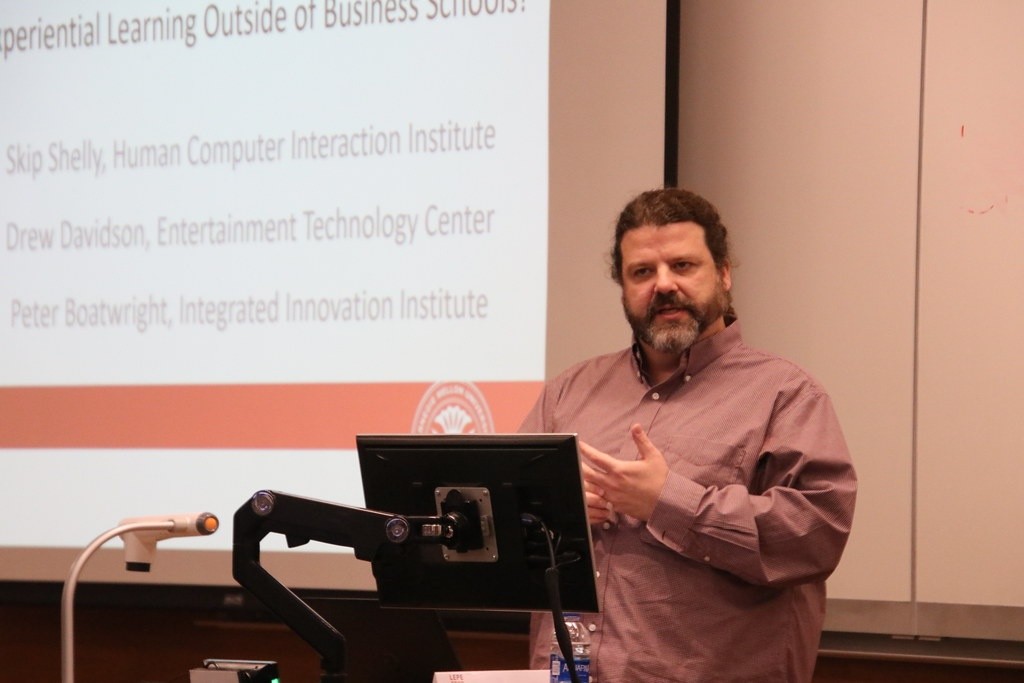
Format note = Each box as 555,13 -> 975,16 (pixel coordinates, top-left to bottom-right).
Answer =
513,187 -> 859,683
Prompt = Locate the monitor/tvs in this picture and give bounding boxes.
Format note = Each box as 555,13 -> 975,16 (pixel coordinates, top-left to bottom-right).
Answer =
357,432 -> 601,615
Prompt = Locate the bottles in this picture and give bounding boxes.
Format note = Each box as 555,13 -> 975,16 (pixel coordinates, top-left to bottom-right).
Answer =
550,612 -> 589,683
